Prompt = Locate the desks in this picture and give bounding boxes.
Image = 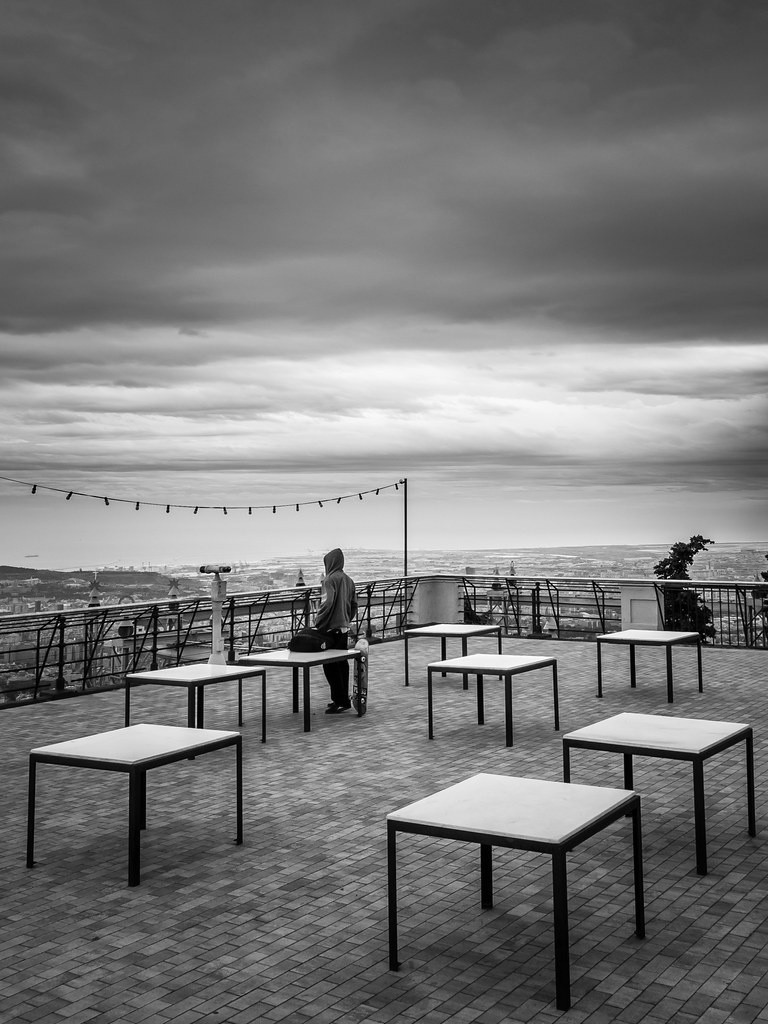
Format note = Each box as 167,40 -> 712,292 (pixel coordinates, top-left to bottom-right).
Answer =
26,723 -> 243,888
563,712 -> 756,875
597,629 -> 703,704
404,623 -> 503,691
238,649 -> 362,733
125,664 -> 268,759
427,654 -> 560,747
386,771 -> 646,1010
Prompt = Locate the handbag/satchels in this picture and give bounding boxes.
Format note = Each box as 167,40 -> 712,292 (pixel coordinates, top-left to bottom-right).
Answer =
287,627 -> 331,653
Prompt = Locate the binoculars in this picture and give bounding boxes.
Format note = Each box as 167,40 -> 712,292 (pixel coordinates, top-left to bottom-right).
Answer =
199,564 -> 232,574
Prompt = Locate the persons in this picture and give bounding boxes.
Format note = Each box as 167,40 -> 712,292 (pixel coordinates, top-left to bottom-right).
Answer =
313,548 -> 360,715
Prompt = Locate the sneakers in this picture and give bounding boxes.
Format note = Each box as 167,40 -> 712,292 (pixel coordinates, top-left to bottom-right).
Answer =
325,699 -> 351,714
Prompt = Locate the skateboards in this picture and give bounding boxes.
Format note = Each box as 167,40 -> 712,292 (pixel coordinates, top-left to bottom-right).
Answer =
348,638 -> 369,714
